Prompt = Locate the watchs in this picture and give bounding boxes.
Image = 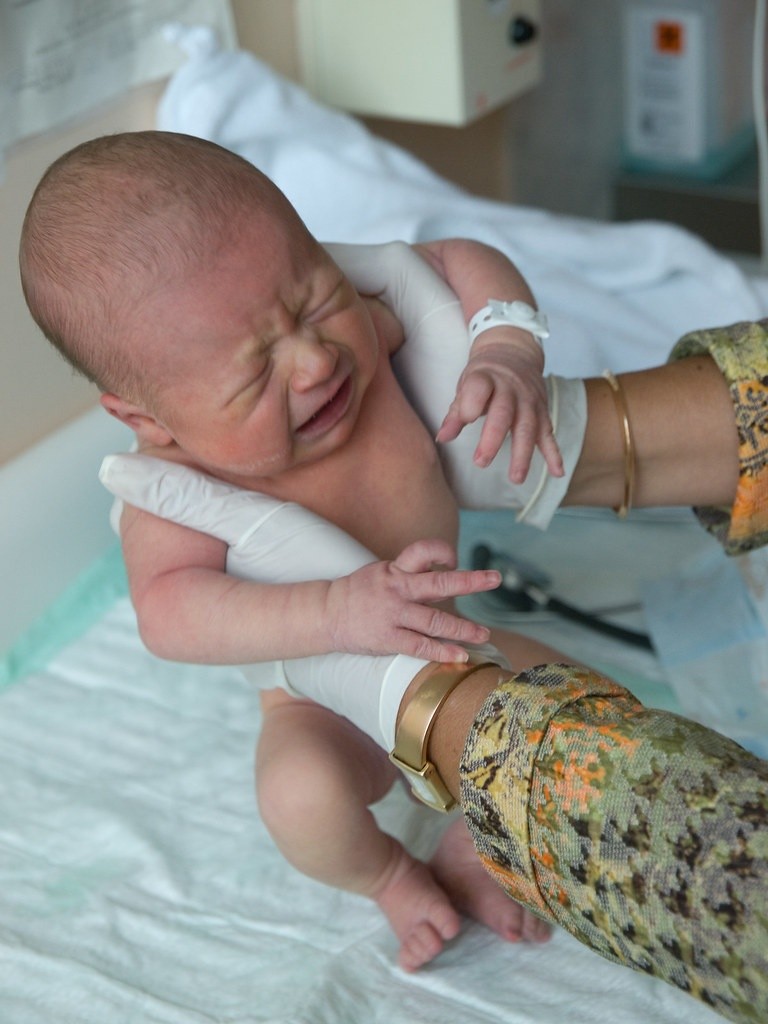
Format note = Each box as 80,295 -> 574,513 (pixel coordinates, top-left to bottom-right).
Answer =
389,654 -> 501,814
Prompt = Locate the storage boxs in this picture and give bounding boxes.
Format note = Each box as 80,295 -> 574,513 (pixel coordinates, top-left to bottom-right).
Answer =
621,0 -> 768,190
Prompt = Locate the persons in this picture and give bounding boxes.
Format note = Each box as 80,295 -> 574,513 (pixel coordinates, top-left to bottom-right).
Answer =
98,241 -> 767,1024
19,131 -> 567,971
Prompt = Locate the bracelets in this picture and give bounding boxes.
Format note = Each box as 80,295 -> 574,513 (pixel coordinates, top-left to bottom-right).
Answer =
602,370 -> 635,517
468,297 -> 549,344
